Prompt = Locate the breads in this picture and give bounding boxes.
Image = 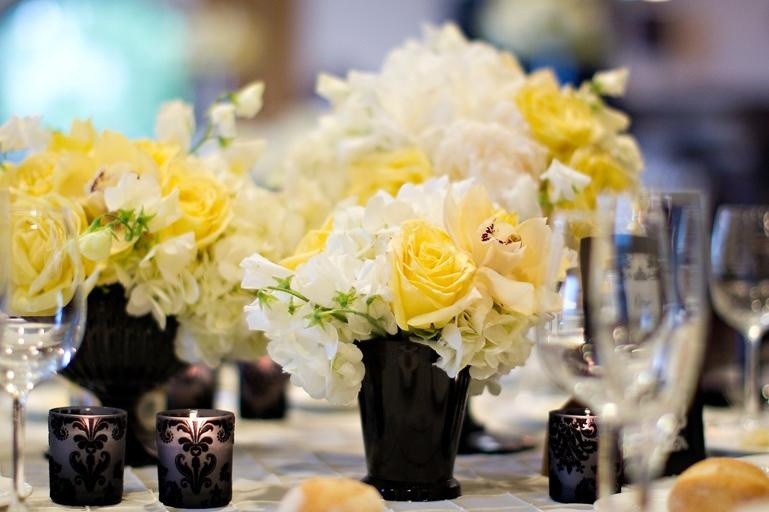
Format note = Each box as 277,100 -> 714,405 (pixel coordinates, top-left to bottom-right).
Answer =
277,474 -> 386,510
666,457 -> 769,511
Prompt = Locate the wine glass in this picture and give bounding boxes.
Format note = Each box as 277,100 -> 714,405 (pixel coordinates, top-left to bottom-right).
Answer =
525,185 -> 710,510
0,204 -> 89,512
708,202 -> 768,417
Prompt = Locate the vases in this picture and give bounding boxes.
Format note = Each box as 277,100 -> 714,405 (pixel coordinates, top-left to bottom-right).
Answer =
49,288 -> 179,468
353,325 -> 473,500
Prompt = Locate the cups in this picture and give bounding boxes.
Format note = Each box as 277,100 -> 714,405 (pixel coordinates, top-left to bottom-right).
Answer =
47,404 -> 129,506
155,408 -> 235,510
547,407 -> 623,505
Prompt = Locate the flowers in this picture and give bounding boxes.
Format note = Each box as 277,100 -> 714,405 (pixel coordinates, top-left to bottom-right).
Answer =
4,21 -> 654,388
